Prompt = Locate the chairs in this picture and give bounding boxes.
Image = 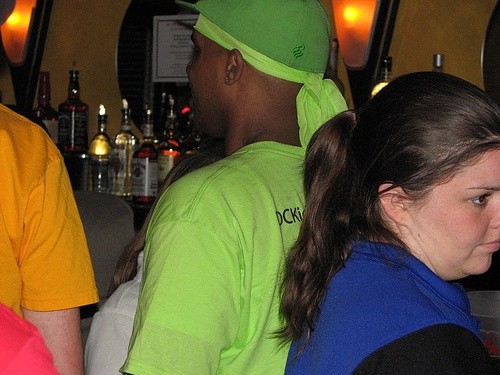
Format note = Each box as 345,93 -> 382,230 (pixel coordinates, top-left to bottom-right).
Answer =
70,190 -> 135,329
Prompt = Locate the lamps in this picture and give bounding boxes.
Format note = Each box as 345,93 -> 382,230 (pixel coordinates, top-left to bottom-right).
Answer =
331,0 -> 400,108
0,0 -> 54,117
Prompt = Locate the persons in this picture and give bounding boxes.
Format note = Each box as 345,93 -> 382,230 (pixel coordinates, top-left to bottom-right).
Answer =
116,0 -> 221,236
0,303 -> 60,375
118,0 -> 348,375
84,155 -> 218,375
270,71 -> 500,375
0,104 -> 101,375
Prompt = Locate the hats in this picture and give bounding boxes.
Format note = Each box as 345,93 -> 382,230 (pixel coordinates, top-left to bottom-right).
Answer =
174,0 -> 331,73
